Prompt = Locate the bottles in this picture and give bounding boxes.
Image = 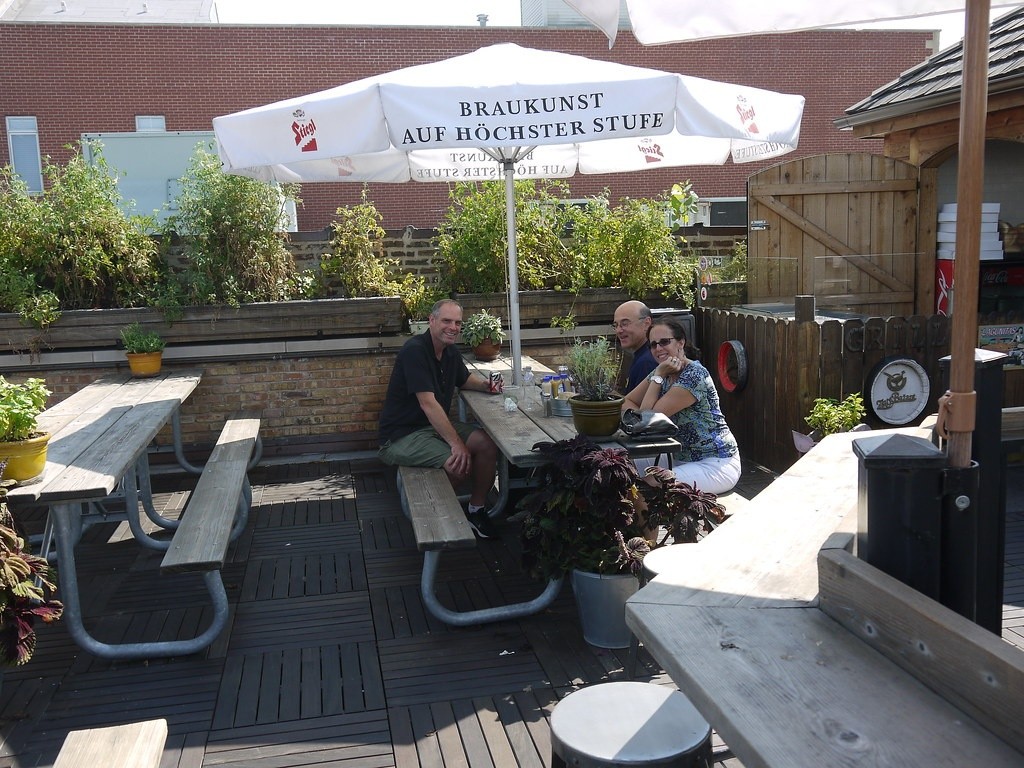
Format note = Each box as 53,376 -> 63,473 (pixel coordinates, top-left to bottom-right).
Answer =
558,366 -> 575,392
511,367 -> 535,411
553,377 -> 563,399
542,375 -> 552,396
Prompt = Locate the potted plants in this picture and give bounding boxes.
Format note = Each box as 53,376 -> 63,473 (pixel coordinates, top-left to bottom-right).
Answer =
427,178 -> 700,332
120,322 -> 168,378
507,437 -> 726,650
0,136 -> 413,349
566,335 -> 625,436
461,307 -> 506,356
0,375 -> 54,482
401,275 -> 450,335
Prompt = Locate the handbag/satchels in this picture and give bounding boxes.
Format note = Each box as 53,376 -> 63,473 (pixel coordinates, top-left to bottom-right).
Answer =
619,407 -> 679,441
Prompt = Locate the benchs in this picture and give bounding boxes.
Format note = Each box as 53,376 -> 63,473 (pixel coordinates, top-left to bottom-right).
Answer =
160,419 -> 261,660
397,465 -> 489,626
709,491 -> 749,523
54,719 -> 168,768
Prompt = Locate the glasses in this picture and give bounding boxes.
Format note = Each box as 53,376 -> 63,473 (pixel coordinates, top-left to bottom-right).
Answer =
610,317 -> 645,330
649,337 -> 679,348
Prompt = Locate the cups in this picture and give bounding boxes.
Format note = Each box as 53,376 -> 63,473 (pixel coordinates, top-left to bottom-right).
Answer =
502,386 -> 520,413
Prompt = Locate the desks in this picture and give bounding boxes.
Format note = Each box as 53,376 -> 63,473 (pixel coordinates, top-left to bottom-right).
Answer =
458,352 -> 682,626
0,370 -> 206,659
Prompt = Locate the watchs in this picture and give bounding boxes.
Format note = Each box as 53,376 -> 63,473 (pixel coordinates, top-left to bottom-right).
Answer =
651,375 -> 664,385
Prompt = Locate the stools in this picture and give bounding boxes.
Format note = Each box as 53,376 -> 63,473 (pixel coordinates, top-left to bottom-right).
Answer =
551,682 -> 716,768
626,542 -> 701,680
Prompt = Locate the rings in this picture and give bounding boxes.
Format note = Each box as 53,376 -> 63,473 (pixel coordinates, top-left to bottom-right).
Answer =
673,359 -> 676,361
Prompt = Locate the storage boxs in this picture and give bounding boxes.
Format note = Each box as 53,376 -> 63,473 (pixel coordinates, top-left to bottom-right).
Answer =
937,203 -> 1003,260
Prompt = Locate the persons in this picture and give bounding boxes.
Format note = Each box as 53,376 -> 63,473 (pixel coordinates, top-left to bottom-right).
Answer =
376,298 -> 505,539
622,315 -> 742,549
611,299 -> 658,392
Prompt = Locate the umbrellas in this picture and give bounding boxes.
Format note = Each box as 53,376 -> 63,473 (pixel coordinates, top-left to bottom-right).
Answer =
207,35 -> 803,384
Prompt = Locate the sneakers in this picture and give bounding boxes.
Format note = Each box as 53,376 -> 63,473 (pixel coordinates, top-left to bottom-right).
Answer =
463,502 -> 496,537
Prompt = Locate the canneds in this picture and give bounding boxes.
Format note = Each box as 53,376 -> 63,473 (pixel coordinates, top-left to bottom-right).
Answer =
557,366 -> 569,375
489,370 -> 501,394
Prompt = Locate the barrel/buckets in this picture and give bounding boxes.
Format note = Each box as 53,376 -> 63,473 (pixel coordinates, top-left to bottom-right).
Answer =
540,392 -> 552,415
550,396 -> 574,416
